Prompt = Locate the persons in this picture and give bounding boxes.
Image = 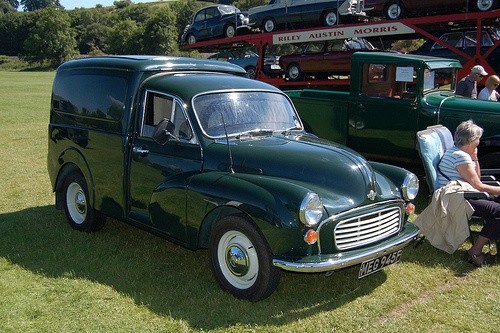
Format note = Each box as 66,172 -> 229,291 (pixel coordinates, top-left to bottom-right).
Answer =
437,120 -> 500,268
455,65 -> 488,97
478,75 -> 500,101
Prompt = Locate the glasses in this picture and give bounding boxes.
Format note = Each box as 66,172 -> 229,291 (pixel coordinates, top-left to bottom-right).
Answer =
494,83 -> 500,86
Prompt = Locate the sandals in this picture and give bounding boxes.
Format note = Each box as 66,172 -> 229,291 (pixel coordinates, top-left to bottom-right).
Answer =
464,250 -> 490,267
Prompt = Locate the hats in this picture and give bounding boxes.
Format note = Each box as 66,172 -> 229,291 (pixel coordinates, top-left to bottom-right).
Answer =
471,65 -> 489,76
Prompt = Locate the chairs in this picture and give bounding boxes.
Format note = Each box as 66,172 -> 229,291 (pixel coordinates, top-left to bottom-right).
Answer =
414,125 -> 500,257
201,100 -> 235,130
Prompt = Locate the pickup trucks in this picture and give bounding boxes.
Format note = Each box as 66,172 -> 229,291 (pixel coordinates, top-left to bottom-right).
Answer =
47,54 -> 425,302
283,52 -> 500,181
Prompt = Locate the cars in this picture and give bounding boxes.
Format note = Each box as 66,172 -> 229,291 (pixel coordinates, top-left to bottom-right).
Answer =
180,0 -> 500,79
